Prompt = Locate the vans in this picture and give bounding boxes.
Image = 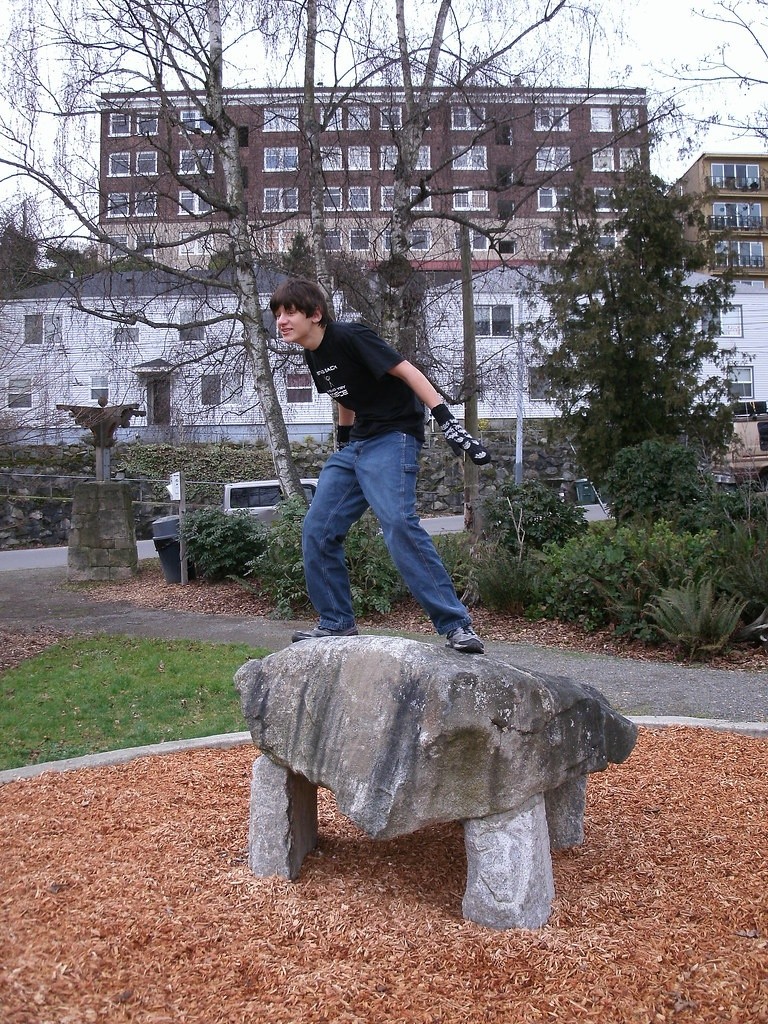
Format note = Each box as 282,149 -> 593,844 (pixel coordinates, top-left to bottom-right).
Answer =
224,478 -> 318,526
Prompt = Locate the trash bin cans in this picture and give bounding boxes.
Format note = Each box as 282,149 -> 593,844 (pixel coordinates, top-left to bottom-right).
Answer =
574,478 -> 596,505
152,515 -> 198,585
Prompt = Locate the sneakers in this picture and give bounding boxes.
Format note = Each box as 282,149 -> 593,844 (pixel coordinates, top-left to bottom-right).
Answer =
445,625 -> 485,654
292,625 -> 359,642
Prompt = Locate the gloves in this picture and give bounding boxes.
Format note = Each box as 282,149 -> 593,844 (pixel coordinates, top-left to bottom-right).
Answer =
336,424 -> 352,451
431,403 -> 491,466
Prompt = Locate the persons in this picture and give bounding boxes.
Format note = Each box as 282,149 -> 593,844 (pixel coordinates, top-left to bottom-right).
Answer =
271,279 -> 494,655
